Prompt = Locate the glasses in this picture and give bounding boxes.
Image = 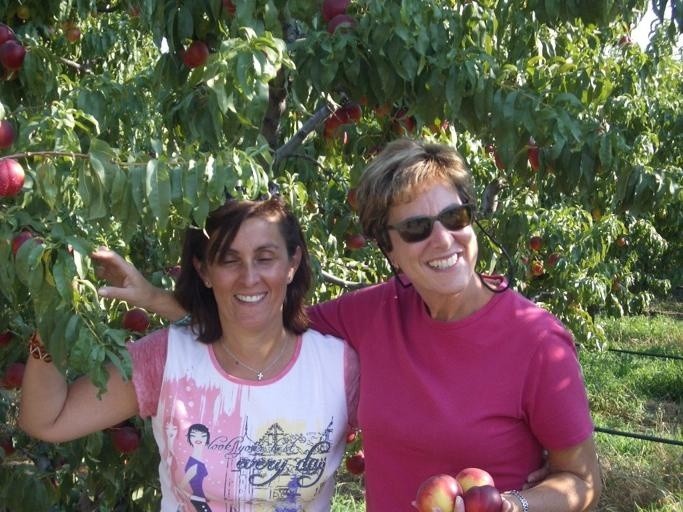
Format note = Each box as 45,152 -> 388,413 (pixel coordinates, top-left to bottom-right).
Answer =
384,202 -> 477,244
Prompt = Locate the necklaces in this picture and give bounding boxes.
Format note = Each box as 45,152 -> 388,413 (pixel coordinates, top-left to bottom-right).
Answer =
217,327 -> 288,380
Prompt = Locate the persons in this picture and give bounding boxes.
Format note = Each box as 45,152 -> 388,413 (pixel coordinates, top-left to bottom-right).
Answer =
88,139 -> 601,512
179,423 -> 214,512
19,198 -> 361,512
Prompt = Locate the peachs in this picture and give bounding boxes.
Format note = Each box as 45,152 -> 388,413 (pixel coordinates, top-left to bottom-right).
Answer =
0,1 -> 634,456
344,454 -> 366,476
415,466 -> 504,512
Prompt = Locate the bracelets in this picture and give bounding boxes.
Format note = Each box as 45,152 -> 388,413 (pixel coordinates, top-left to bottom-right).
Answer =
27,334 -> 53,362
172,312 -> 193,325
505,489 -> 529,512
